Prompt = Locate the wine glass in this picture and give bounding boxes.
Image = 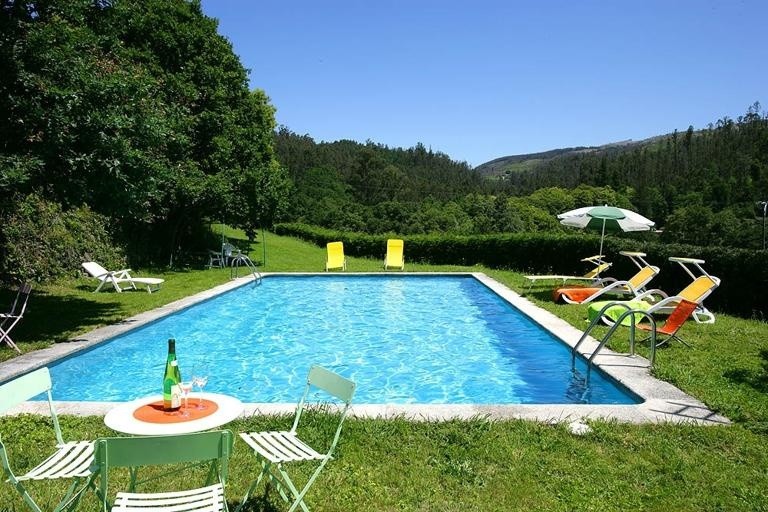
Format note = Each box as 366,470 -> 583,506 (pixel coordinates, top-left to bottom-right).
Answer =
178,366 -> 194,418
194,364 -> 209,411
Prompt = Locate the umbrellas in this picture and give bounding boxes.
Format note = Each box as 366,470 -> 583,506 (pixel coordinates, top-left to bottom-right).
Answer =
556,203 -> 656,278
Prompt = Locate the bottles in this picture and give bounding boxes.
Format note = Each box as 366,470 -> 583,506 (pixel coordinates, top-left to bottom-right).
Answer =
164,338 -> 181,411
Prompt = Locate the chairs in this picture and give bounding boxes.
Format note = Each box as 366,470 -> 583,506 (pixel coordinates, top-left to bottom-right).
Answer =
521,251 -> 722,349
205,242 -> 242,269
82,261 -> 164,294
0,284 -> 33,353
383,239 -> 405,271
325,241 -> 346,272
